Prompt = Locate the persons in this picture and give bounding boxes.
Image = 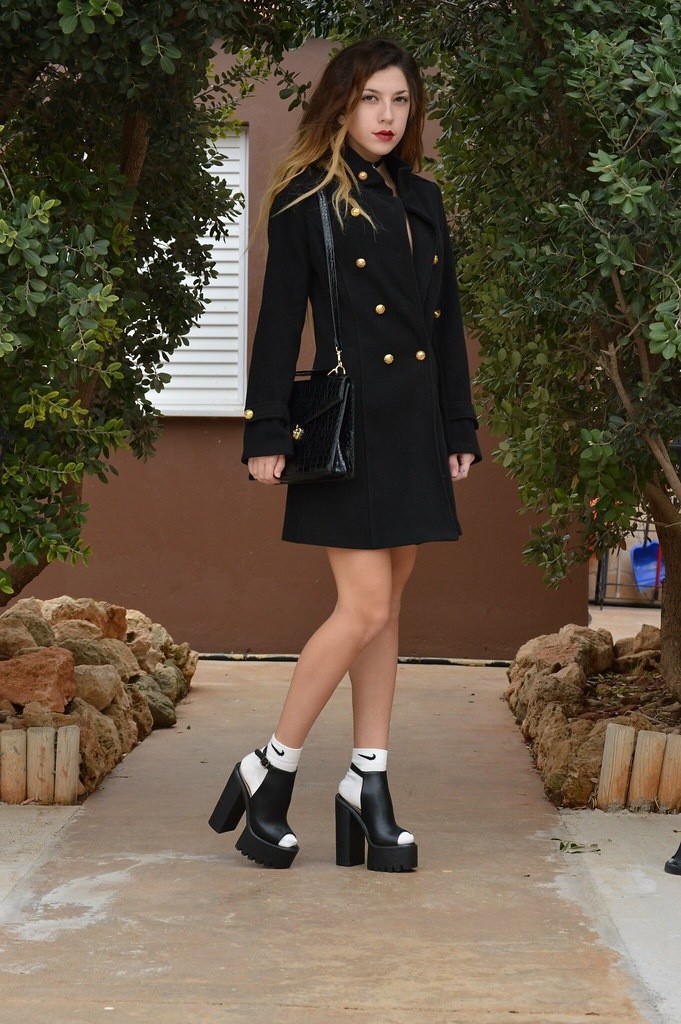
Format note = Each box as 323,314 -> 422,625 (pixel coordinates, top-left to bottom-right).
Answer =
208,38 -> 482,873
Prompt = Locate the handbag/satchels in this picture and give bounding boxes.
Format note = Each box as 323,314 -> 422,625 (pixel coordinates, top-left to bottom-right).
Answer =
249,372 -> 355,483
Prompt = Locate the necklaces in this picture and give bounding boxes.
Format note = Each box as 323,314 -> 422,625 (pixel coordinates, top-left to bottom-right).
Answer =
373,155 -> 386,168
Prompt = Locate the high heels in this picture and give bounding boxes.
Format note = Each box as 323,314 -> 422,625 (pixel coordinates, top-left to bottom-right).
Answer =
207,746 -> 300,869
334,763 -> 419,872
